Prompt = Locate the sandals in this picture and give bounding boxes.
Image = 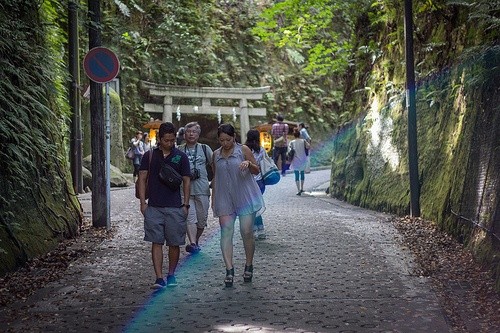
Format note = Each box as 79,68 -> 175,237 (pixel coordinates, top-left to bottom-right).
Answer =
242,264 -> 253,283
224,266 -> 234,287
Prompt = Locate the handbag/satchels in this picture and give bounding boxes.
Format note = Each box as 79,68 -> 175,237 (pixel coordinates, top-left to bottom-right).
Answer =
259,146 -> 281,185
202,144 -> 213,181
135,148 -> 150,200
125,141 -> 136,160
287,143 -> 295,163
273,135 -> 285,148
158,161 -> 184,191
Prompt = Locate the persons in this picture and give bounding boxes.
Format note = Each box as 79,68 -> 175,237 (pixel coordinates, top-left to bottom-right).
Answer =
130,130 -> 156,183
174,127 -> 187,147
244,129 -> 269,232
176,122 -> 214,255
212,124 -> 266,286
289,129 -> 310,195
299,122 -> 311,174
271,114 -> 288,176
138,121 -> 192,288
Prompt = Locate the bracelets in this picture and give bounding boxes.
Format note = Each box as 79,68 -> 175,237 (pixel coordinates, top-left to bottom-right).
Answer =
139,203 -> 147,205
182,204 -> 190,209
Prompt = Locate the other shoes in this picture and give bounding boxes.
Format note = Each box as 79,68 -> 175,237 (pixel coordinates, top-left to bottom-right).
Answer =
166,275 -> 178,287
296,190 -> 303,196
152,277 -> 166,289
185,243 -> 199,254
255,231 -> 266,241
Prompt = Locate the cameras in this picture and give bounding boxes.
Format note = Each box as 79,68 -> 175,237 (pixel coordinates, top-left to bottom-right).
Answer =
191,168 -> 200,182
